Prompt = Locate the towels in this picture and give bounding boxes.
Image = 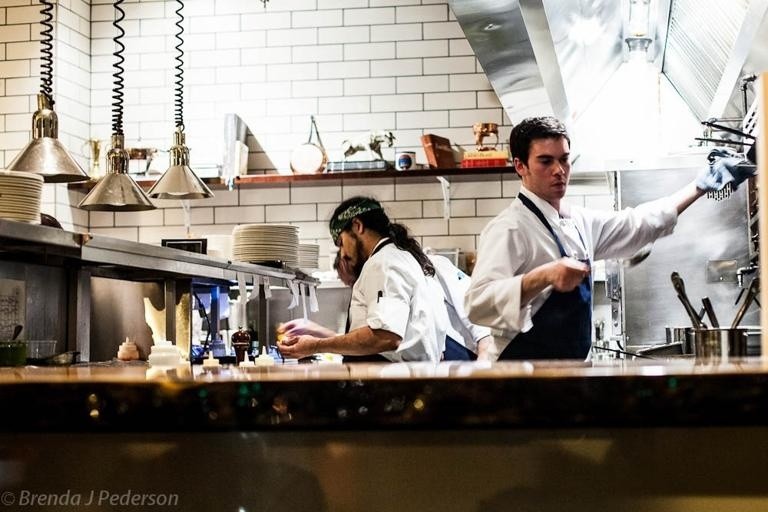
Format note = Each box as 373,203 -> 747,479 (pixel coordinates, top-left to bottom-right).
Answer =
696,146 -> 752,192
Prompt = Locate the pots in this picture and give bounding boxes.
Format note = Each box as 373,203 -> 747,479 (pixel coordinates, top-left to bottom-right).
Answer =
289,116 -> 327,173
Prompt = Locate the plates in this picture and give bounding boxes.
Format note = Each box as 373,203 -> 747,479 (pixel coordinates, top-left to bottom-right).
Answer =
0,168 -> 45,225
232,223 -> 299,263
298,244 -> 320,270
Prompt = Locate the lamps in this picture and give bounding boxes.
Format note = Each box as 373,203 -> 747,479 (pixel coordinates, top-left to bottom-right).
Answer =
76,0 -> 157,213
147,1 -> 216,200
6,1 -> 91,184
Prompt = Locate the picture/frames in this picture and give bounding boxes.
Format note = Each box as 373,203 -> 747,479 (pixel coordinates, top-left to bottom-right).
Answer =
161,238 -> 208,255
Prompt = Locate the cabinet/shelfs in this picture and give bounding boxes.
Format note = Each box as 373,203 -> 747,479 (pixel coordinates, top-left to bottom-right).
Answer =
1,357 -> 767,510
0,219 -> 321,367
606,170 -> 760,359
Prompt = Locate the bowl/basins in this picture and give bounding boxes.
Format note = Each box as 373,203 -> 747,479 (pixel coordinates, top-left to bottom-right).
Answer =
684,327 -> 747,357
664,325 -> 706,343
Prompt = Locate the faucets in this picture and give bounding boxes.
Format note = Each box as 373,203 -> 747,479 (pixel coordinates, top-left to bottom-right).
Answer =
736,265 -> 760,286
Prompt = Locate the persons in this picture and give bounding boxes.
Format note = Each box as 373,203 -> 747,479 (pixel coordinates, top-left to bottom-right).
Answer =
465,117 -> 712,358
280,198 -> 437,363
277,222 -> 497,362
